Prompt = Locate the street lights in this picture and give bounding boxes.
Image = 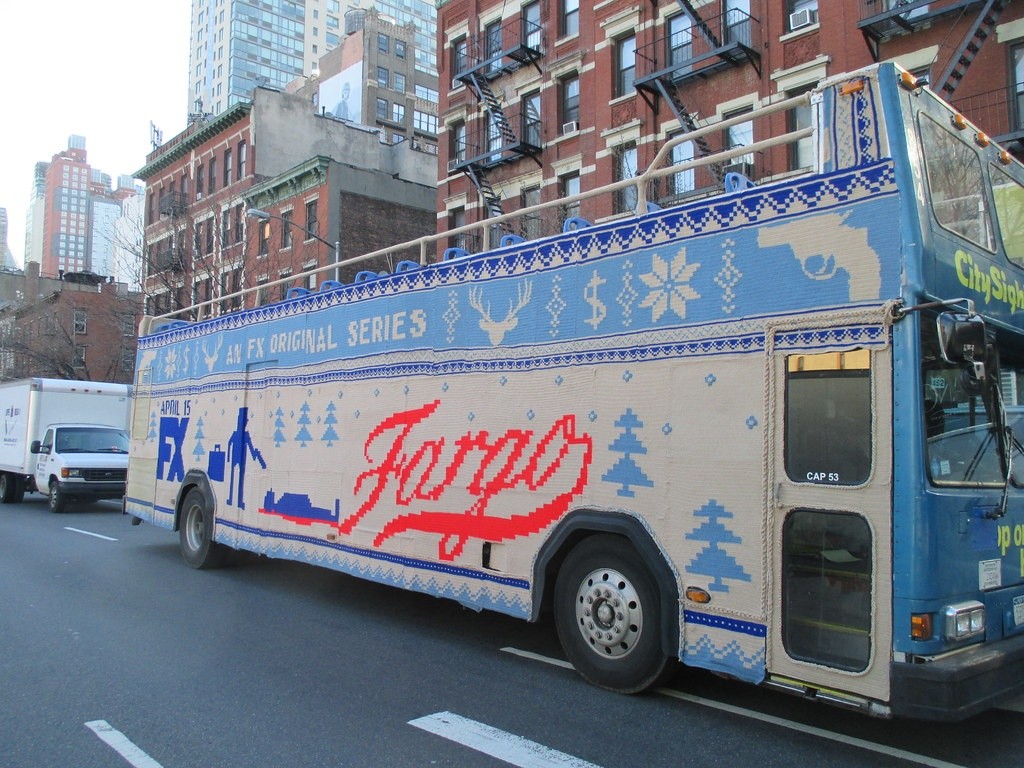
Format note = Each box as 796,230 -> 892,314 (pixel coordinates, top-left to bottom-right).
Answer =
246,207 -> 340,286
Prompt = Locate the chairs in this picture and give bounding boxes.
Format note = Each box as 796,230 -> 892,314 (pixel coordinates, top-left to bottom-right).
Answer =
443,248 -> 471,262
319,280 -> 344,293
49,435 -> 70,451
396,260 -> 420,274
286,287 -> 311,299
725,171 -> 757,193
168,321 -> 188,329
354,270 -> 380,283
158,324 -> 177,332
499,235 -> 528,247
635,200 -> 664,217
563,217 -> 592,233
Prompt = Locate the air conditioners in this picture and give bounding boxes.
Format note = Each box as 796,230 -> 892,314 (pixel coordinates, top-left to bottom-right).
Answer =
452,78 -> 465,90
196,192 -> 205,200
721,162 -> 751,181
562,121 -> 579,135
447,159 -> 463,174
193,250 -> 200,258
790,9 -> 814,31
526,45 -> 539,57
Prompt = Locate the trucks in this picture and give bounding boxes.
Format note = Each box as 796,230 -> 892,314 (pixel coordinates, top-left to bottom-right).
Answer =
0,375 -> 134,514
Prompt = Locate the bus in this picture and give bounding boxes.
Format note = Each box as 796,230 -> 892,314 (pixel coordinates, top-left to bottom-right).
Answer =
121,58 -> 1023,729
